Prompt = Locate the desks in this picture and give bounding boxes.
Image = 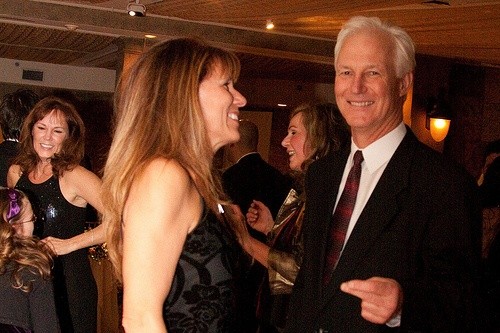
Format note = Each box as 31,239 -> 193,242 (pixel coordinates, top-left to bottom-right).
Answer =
87,252 -> 121,333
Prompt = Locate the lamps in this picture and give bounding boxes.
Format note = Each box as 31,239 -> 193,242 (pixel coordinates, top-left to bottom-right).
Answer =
426,107 -> 451,141
266,19 -> 274,29
127,0 -> 147,18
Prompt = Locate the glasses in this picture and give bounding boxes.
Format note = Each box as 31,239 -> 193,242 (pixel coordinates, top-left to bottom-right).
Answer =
13,213 -> 39,226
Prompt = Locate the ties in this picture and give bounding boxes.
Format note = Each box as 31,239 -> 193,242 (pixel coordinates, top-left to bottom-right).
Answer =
323,150 -> 365,294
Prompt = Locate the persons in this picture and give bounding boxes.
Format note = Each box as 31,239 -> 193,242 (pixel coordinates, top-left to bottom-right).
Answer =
284,17 -> 484,333
223,101 -> 351,296
223,120 -> 292,245
0,89 -> 106,333
97,34 -> 267,333
